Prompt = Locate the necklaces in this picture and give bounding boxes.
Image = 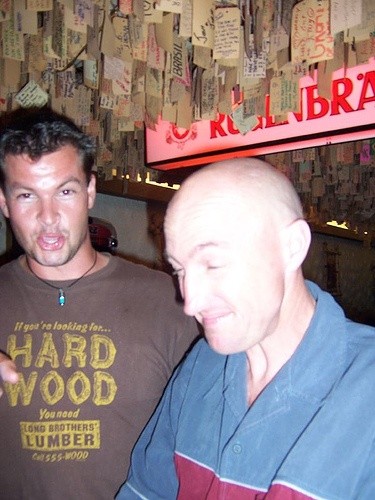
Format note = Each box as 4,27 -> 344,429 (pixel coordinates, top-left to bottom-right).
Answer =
25,251 -> 99,306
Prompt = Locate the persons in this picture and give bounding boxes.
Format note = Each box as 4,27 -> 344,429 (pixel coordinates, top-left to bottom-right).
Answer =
110,158 -> 375,500
2,104 -> 205,500
